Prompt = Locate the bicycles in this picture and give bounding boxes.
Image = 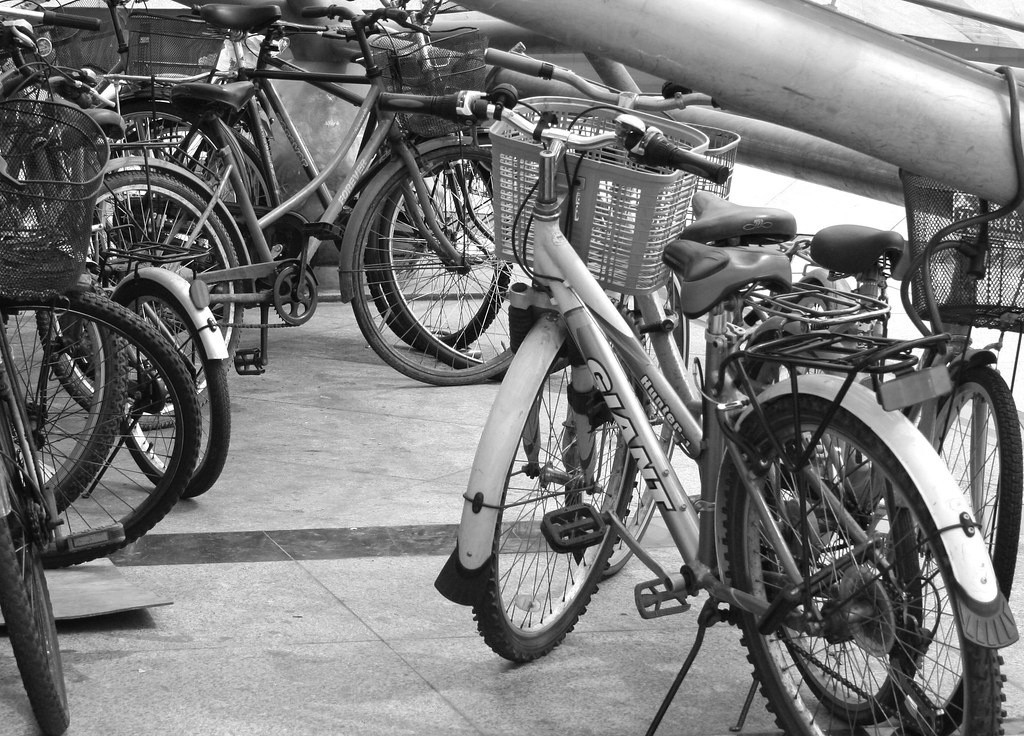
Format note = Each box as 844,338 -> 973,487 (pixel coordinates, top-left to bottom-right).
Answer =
0,2 -> 1024,736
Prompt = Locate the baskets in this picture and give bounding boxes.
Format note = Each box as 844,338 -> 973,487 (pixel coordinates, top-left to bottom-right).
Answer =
489,96 -> 709,293
127,11 -> 227,84
370,27 -> 487,138
11,0 -> 121,74
681,122 -> 742,229
0,23 -> 82,77
0,98 -> 110,302
899,167 -> 1024,333
